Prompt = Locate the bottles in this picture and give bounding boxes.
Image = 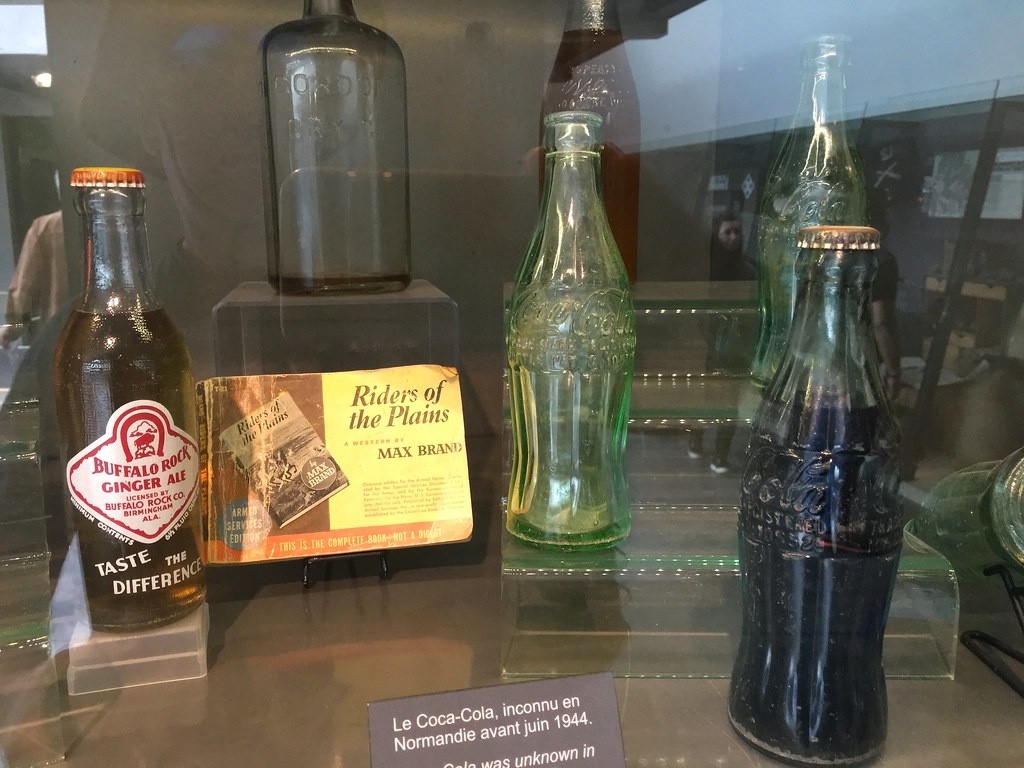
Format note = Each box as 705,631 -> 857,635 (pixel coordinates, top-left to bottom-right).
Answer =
749,34 -> 872,398
50,167 -> 207,633
504,112 -> 637,554
903,446 -> 1023,590
726,225 -> 903,768
256,0 -> 414,294
539,0 -> 641,288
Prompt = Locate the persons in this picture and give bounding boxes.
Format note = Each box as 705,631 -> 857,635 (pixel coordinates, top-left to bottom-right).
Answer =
0,169 -> 67,597
864,213 -> 901,399
685,212 -> 773,475
